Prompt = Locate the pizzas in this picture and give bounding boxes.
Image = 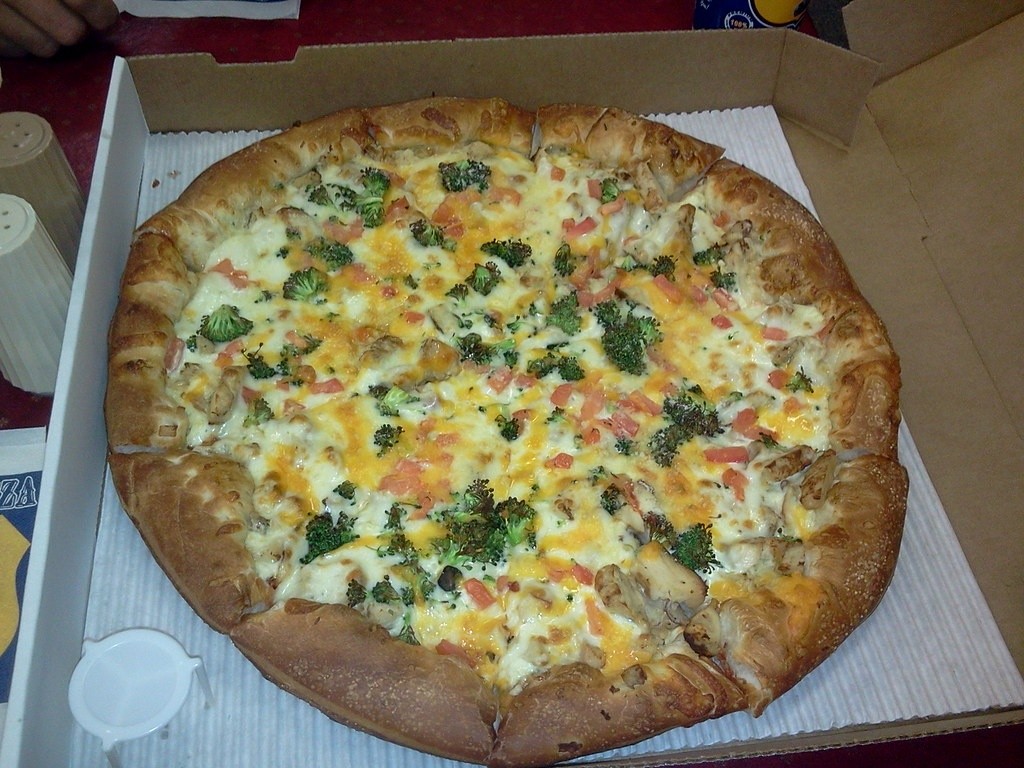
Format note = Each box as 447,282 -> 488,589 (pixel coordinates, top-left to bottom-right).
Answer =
104,97 -> 908,767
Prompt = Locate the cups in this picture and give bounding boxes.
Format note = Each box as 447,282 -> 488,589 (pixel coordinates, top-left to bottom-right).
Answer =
0,193 -> 74,394
0,112 -> 86,275
